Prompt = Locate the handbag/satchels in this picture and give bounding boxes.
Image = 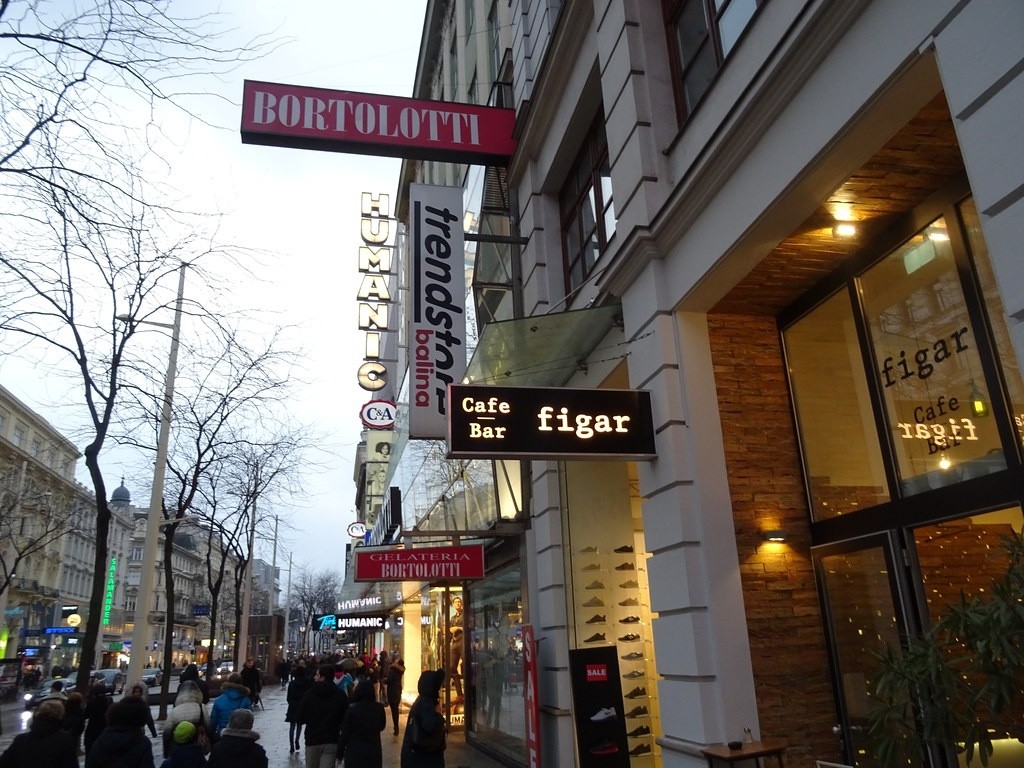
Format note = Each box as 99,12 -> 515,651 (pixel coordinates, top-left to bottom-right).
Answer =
198,703 -> 211,755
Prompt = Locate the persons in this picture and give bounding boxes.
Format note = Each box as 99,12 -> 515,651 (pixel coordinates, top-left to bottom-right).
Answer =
400,668 -> 446,768
0,650 -> 405,768
449,597 -> 465,696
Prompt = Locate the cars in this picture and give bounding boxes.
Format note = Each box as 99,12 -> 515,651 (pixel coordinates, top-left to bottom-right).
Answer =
67,670 -> 105,694
24,677 -> 77,710
180,662 -> 204,678
144,667 -> 164,687
217,662 -> 234,673
201,663 -> 217,675
102,669 -> 125,695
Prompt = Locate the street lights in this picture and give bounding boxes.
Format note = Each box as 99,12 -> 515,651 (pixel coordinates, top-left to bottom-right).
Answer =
299,627 -> 306,631
180,643 -> 192,648
152,643 -> 157,650
278,644 -> 284,650
224,490 -> 256,675
274,567 -> 291,662
291,587 -> 308,652
112,314 -> 181,697
15,650 -> 26,656
254,536 -> 276,681
10,567 -> 17,578
231,631 -> 237,637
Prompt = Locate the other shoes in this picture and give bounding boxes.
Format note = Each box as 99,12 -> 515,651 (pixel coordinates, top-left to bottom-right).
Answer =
289,742 -> 294,753
295,738 -> 300,750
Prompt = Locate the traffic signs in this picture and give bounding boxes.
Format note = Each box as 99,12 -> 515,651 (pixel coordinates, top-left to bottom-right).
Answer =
192,604 -> 210,616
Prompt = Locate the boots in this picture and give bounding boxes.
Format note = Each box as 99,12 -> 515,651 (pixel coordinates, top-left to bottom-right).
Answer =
393,727 -> 399,735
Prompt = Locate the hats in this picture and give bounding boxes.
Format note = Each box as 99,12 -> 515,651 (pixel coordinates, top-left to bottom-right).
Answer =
173,720 -> 196,743
418,668 -> 445,696
49,681 -> 64,690
